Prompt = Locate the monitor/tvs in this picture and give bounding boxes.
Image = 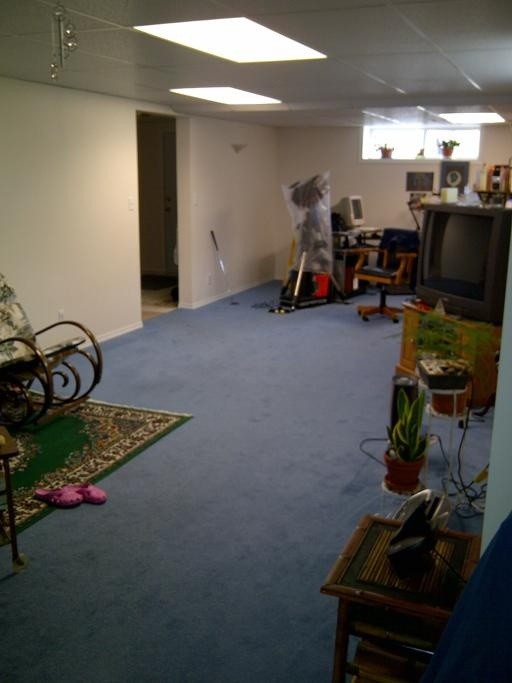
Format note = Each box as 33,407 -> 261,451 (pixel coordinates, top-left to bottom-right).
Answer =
415,204 -> 512,327
331,195 -> 365,229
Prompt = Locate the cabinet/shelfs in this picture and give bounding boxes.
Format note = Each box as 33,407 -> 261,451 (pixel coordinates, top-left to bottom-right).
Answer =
393,299 -> 501,418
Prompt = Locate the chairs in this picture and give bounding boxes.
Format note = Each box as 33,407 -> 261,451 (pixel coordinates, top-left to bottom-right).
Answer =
1,273 -> 103,433
352,228 -> 418,323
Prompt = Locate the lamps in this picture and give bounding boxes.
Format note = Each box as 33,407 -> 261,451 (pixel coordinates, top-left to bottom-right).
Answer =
49,0 -> 79,83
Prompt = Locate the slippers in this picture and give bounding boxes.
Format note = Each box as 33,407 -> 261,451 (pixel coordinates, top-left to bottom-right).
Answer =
56,482 -> 107,504
34,485 -> 84,507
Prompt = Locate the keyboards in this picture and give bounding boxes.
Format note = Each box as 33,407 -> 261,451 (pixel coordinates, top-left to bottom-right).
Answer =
356,227 -> 380,232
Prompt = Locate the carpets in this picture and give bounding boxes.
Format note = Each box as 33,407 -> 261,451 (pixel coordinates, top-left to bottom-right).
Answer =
1,386 -> 194,545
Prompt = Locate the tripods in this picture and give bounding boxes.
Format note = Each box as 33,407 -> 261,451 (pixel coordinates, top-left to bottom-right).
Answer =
280,207 -> 352,311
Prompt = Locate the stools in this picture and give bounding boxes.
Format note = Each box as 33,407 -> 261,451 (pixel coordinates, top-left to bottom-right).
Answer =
0,425 -> 23,564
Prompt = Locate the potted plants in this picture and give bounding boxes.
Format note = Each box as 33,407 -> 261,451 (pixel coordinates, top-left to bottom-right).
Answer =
384,388 -> 430,491
373,139 -> 459,158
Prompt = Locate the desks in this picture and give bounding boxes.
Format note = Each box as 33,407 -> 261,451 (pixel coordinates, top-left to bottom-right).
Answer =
333,226 -> 382,299
321,514 -> 480,682
